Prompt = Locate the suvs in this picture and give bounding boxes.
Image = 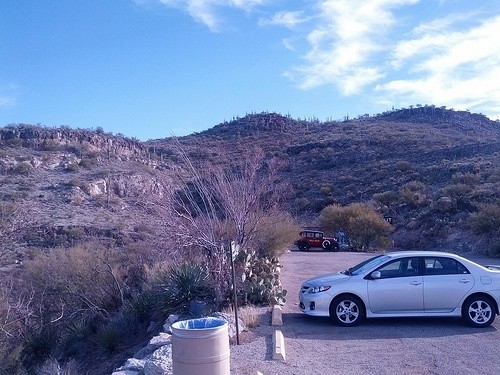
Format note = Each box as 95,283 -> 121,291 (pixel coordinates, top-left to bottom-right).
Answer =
296,230 -> 337,251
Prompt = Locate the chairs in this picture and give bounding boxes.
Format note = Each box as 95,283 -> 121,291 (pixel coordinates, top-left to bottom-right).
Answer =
435,260 -> 460,274
403,258 -> 426,276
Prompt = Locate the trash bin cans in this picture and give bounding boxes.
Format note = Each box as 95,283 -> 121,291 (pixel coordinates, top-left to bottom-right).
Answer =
171,317 -> 231,375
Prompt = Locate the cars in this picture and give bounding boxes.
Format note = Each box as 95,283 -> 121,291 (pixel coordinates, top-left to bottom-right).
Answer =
299,251 -> 500,328
